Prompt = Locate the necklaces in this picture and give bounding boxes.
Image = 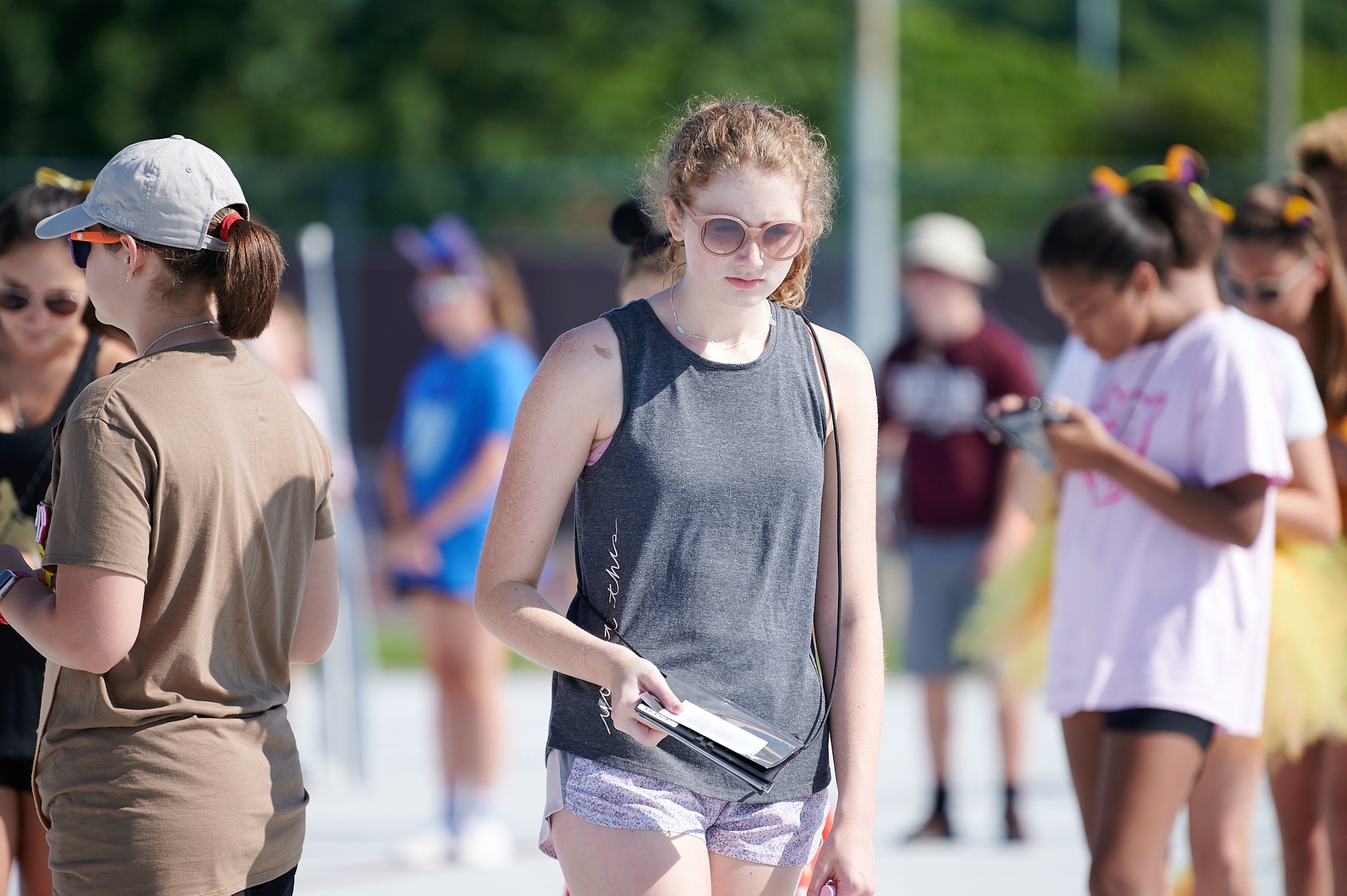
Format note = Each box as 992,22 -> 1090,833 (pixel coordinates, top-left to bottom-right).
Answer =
142,320 -> 219,357
5,362 -> 70,430
670,276 -> 769,351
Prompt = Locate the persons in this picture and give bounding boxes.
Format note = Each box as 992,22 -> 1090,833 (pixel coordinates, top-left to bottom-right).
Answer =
948,110 -> 1347,895
0,112 -> 342,896
387,216 -> 544,869
469,100 -> 885,896
608,196 -> 687,307
0,177 -> 142,896
870,211 -> 1047,857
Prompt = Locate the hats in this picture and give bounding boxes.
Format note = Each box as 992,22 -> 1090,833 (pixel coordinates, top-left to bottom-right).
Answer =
901,212 -> 1003,290
34,134 -> 250,253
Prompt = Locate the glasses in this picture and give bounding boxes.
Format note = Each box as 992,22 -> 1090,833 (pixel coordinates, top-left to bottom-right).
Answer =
1224,252 -> 1313,301
677,196 -> 814,260
0,285 -> 79,317
69,228 -> 124,267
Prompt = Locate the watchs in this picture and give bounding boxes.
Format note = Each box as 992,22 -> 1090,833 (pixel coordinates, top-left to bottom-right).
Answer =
0,569 -> 36,625
